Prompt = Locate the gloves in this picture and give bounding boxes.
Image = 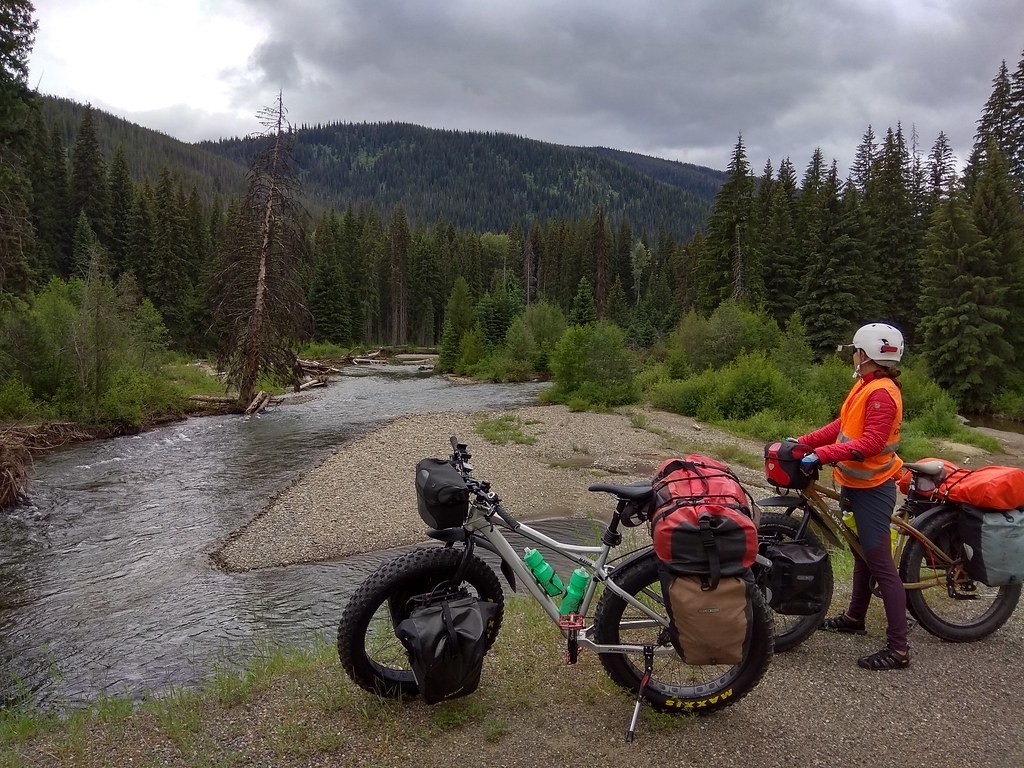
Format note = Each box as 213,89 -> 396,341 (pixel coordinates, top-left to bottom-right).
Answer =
786,437 -> 799,443
799,452 -> 819,475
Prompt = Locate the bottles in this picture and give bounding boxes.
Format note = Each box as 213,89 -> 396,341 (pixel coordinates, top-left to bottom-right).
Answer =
559,565 -> 591,616
523,546 -> 563,596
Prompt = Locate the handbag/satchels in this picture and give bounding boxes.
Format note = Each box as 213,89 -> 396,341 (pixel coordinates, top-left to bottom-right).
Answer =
956,505 -> 1024,588
657,566 -> 755,665
395,596 -> 498,705
621,453 -> 758,592
756,543 -> 831,615
897,457 -> 1024,510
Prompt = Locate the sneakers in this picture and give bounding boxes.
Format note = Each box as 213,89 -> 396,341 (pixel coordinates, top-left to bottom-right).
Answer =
858,645 -> 910,670
818,608 -> 866,634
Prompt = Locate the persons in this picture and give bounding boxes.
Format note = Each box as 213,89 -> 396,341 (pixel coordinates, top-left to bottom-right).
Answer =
785,324 -> 910,671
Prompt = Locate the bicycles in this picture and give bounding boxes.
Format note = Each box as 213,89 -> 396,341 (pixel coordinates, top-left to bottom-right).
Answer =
749,438 -> 1022,655
335,437 -> 775,744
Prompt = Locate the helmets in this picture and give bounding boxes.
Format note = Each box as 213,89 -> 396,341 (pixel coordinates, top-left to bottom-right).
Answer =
853,323 -> 905,367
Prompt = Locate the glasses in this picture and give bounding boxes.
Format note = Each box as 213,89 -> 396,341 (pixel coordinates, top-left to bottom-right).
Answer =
853,346 -> 857,354
